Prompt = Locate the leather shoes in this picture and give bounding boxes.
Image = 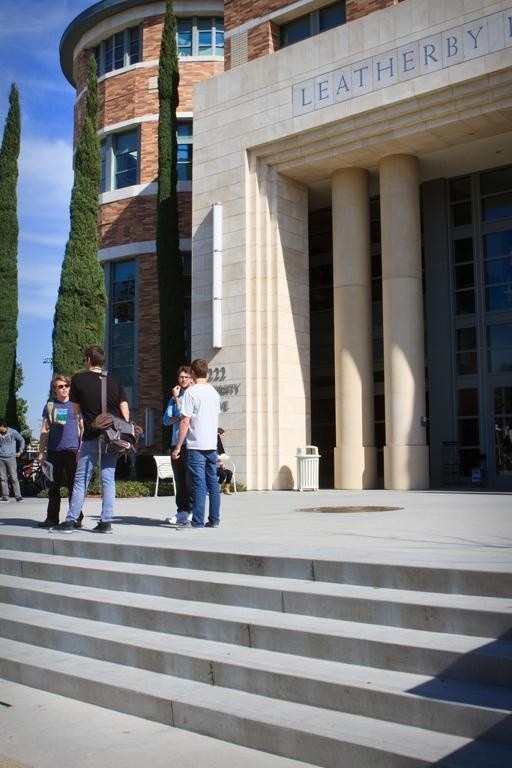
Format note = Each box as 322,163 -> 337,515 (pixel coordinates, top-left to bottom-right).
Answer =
205,522 -> 219,527
176,521 -> 203,530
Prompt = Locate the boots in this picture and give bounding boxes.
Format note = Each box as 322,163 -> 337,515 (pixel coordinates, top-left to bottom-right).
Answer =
218,483 -> 232,495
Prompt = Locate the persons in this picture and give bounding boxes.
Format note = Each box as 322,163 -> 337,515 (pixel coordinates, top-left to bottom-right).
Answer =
216,426 -> 235,495
170,357 -> 221,530
0,418 -> 26,504
161,365 -> 195,529
36,373 -> 85,530
47,345 -> 130,534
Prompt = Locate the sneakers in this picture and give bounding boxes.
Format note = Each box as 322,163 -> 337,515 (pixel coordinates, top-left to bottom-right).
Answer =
166,511 -> 192,524
39,518 -> 60,527
0,496 -> 10,502
93,519 -> 113,532
49,519 -> 83,533
15,497 -> 24,502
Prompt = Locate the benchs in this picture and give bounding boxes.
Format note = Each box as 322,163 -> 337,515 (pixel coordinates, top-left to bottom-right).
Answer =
152,454 -> 237,497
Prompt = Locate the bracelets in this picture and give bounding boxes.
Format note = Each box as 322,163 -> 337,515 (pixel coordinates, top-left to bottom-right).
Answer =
175,396 -> 179,399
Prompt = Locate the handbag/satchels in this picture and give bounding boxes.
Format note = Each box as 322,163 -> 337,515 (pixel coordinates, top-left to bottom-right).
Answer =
91,413 -> 143,457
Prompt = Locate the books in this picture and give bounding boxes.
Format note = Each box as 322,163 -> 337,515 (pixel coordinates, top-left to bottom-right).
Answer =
41,460 -> 54,482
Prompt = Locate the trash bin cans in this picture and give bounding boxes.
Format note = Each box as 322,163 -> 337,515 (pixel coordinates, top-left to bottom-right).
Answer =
295,445 -> 322,492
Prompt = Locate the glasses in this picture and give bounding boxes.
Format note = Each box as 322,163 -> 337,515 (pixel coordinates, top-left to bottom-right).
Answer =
54,384 -> 71,388
